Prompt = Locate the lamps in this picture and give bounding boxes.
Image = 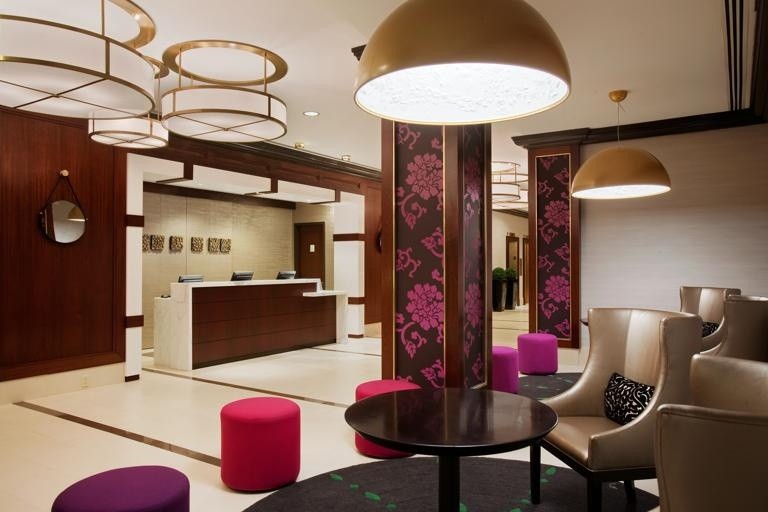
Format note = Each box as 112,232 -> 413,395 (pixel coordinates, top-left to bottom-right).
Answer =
0,0 -> 288,149
67,207 -> 85,221
353,0 -> 571,126
492,161 -> 528,209
571,90 -> 672,199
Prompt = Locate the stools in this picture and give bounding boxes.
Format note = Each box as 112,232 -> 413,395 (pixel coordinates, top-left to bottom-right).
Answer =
518,333 -> 558,375
355,380 -> 422,459
51,466 -> 189,512
221,397 -> 300,493
492,346 -> 519,393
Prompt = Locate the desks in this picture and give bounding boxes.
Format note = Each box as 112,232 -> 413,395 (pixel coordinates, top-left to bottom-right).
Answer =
344,387 -> 559,512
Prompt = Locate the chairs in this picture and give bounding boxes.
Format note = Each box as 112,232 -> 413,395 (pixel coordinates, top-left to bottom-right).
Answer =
703,294 -> 768,360
535,305 -> 703,505
678,286 -> 741,349
657,351 -> 767,512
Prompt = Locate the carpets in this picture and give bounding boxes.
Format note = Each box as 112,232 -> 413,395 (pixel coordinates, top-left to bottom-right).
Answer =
242,457 -> 659,512
517,373 -> 582,400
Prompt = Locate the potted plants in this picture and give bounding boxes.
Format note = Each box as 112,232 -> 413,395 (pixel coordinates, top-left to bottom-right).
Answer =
505,268 -> 519,310
492,268 -> 508,312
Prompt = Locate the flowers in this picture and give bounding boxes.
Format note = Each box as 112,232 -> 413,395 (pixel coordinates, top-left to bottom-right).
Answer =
537,155 -> 570,338
396,123 -> 485,388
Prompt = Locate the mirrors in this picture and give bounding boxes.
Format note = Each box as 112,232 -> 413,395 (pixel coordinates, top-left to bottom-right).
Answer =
40,169 -> 89,246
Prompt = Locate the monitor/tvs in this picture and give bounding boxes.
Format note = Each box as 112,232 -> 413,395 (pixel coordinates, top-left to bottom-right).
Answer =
276,271 -> 296,279
231,271 -> 254,281
179,275 -> 204,282
